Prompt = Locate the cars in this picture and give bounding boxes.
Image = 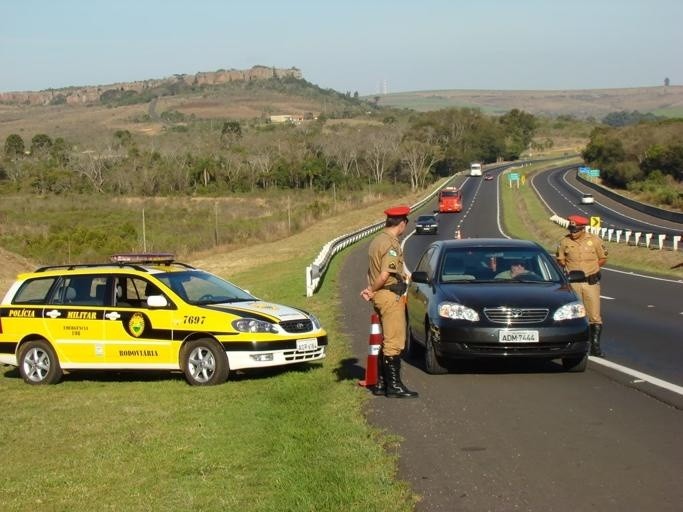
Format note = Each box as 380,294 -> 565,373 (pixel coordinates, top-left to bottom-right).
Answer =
414,214 -> 440,234
405,239 -> 588,375
581,194 -> 594,204
484,172 -> 493,180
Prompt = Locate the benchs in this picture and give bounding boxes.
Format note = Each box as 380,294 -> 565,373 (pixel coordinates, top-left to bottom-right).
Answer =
59,284 -> 106,306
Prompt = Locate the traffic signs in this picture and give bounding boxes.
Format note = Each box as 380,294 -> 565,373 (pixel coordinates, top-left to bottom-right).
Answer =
588,169 -> 599,176
506,172 -> 520,179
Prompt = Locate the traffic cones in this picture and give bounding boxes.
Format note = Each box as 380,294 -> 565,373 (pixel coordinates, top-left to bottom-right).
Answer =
358,314 -> 384,389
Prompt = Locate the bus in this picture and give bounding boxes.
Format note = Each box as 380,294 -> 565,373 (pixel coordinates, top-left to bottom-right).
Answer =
435,186 -> 462,213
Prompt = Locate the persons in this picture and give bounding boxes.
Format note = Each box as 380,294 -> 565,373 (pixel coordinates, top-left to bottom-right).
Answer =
554,215 -> 608,358
494,262 -> 536,280
358,206 -> 419,399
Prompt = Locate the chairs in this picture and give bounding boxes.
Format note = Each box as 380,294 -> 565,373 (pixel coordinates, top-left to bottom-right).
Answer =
441,258 -> 476,281
115,284 -> 133,308
141,282 -> 163,308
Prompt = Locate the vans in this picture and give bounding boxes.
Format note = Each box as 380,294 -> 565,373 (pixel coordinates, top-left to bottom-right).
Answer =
0,253 -> 326,385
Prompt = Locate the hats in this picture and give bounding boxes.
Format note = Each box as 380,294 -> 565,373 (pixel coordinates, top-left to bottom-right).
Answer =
384,207 -> 410,222
567,215 -> 590,230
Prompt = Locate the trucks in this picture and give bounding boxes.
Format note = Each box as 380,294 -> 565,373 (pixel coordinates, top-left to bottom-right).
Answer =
469,163 -> 482,177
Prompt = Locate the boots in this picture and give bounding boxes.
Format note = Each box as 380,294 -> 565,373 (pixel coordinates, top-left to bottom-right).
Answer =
374,356 -> 418,397
590,324 -> 608,357
